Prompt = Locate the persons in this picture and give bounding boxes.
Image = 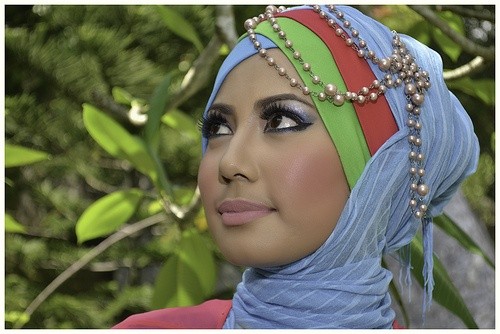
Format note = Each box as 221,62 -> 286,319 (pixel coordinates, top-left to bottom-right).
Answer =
104,5 -> 481,330
